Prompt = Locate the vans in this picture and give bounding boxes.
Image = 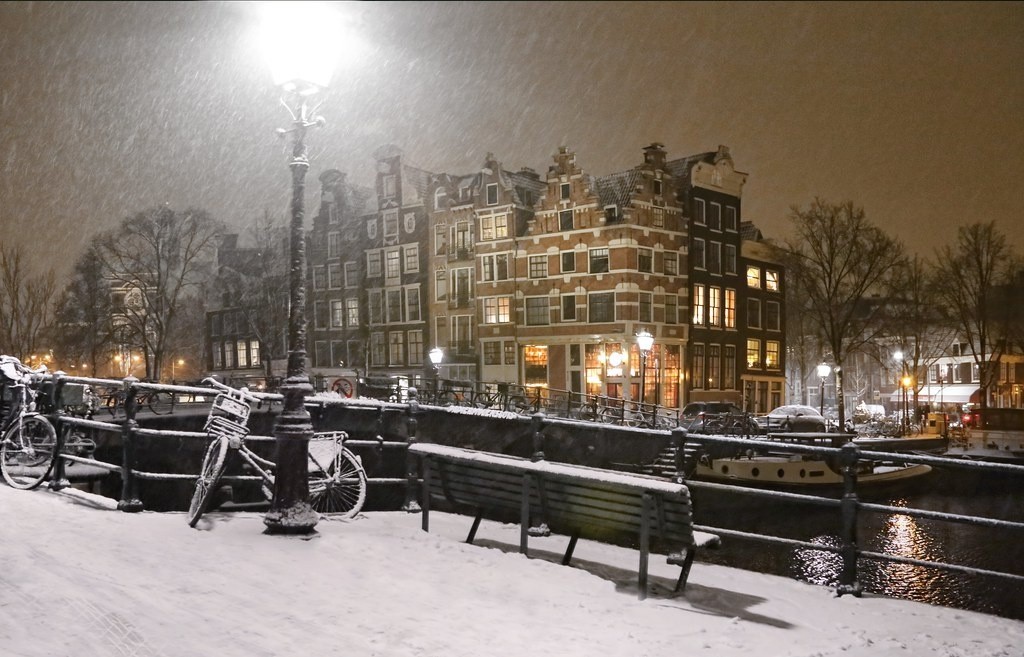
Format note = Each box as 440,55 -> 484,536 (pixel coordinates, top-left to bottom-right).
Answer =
679,400 -> 759,436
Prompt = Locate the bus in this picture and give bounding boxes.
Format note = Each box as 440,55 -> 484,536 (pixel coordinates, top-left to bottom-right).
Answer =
968,408 -> 1024,456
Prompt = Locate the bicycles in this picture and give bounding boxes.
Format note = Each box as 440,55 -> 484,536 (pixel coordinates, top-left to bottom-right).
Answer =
0,354 -> 58,490
187,376 -> 368,528
106,379 -> 174,421
418,379 -> 759,439
859,412 -> 919,439
817,362 -> 832,416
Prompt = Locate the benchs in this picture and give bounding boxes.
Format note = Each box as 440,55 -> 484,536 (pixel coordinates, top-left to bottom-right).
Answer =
405,444 -> 721,601
767,415 -> 857,433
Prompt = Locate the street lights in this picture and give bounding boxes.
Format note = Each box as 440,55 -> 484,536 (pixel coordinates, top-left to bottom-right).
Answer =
427,346 -> 444,403
261,35 -> 325,535
636,331 -> 654,410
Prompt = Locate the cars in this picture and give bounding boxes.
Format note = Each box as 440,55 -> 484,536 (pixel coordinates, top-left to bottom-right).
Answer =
756,404 -> 832,436
